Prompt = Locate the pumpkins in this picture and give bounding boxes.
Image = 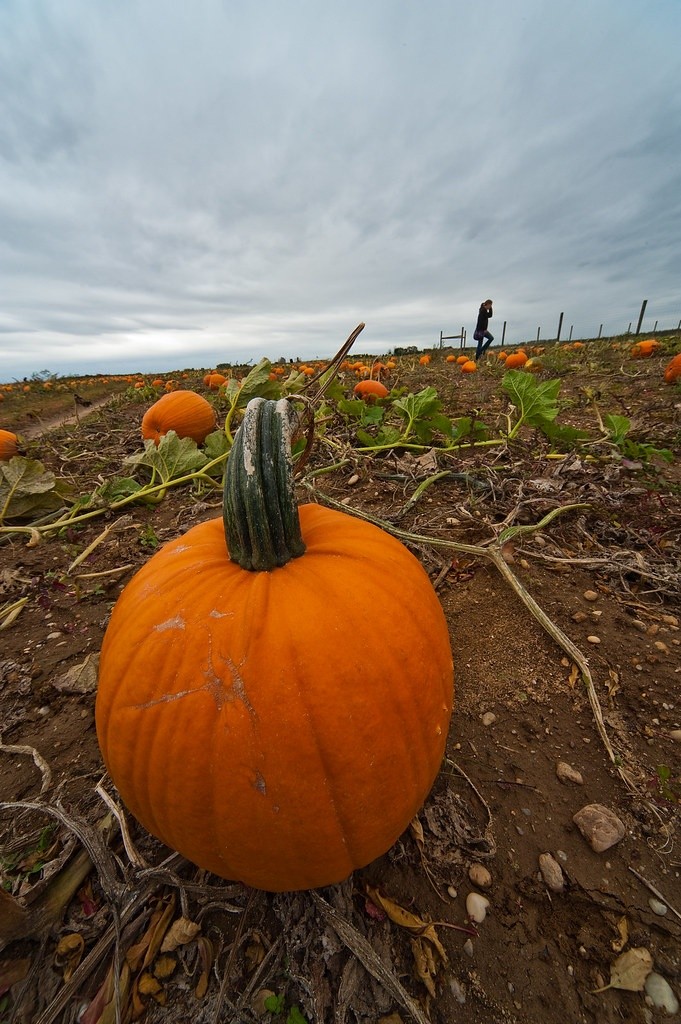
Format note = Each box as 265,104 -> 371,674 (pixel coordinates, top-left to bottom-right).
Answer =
95,398 -> 453,891
0,339 -> 681,460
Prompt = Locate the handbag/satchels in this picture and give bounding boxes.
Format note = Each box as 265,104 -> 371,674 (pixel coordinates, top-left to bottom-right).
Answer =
474,329 -> 483,340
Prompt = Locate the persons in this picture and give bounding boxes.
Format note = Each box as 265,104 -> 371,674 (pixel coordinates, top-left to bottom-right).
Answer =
473,299 -> 494,361
